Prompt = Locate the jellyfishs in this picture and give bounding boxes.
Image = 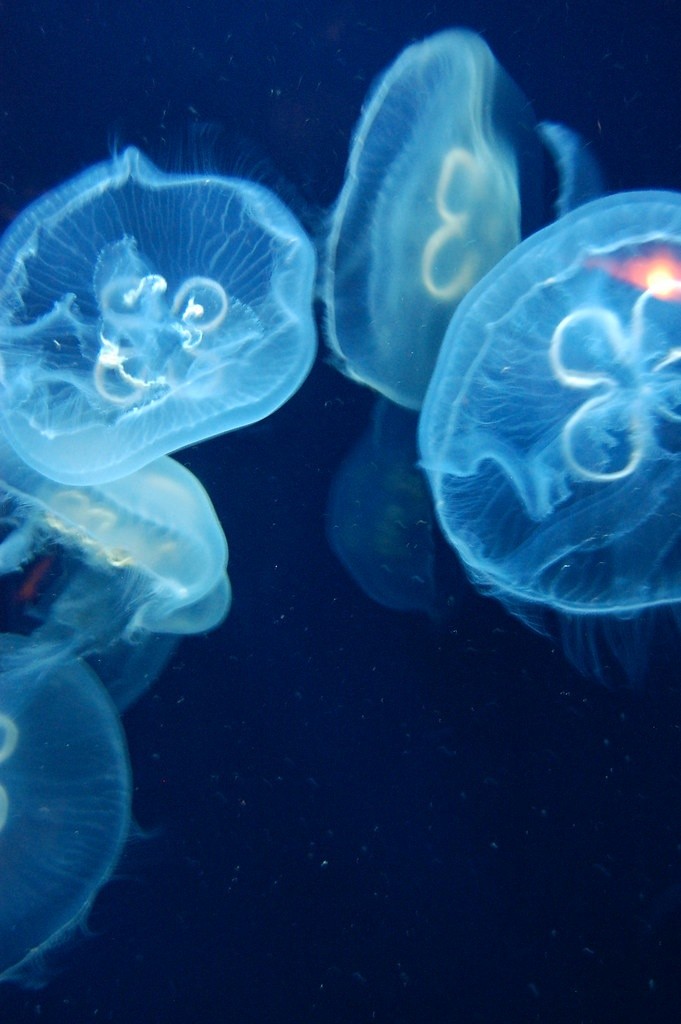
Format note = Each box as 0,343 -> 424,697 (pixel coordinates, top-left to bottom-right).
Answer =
0,25 -> 681,981
